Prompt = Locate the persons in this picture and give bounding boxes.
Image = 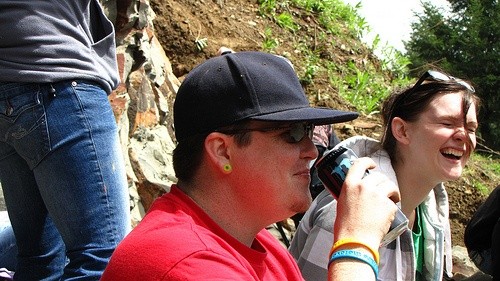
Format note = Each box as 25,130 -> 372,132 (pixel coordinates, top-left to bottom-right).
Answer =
289,70 -> 478,281
464,185 -> 500,281
0,0 -> 399,281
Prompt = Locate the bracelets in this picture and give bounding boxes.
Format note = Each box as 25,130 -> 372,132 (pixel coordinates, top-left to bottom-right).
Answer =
328,236 -> 380,281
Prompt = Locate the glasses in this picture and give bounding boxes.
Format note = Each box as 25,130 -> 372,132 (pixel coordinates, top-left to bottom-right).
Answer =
406,70 -> 476,101
224,121 -> 315,144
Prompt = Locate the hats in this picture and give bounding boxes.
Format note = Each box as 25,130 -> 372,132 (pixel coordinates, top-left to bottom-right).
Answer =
173,50 -> 360,142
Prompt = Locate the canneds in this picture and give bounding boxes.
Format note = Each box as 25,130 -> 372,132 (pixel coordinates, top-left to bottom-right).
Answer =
314,146 -> 409,247
